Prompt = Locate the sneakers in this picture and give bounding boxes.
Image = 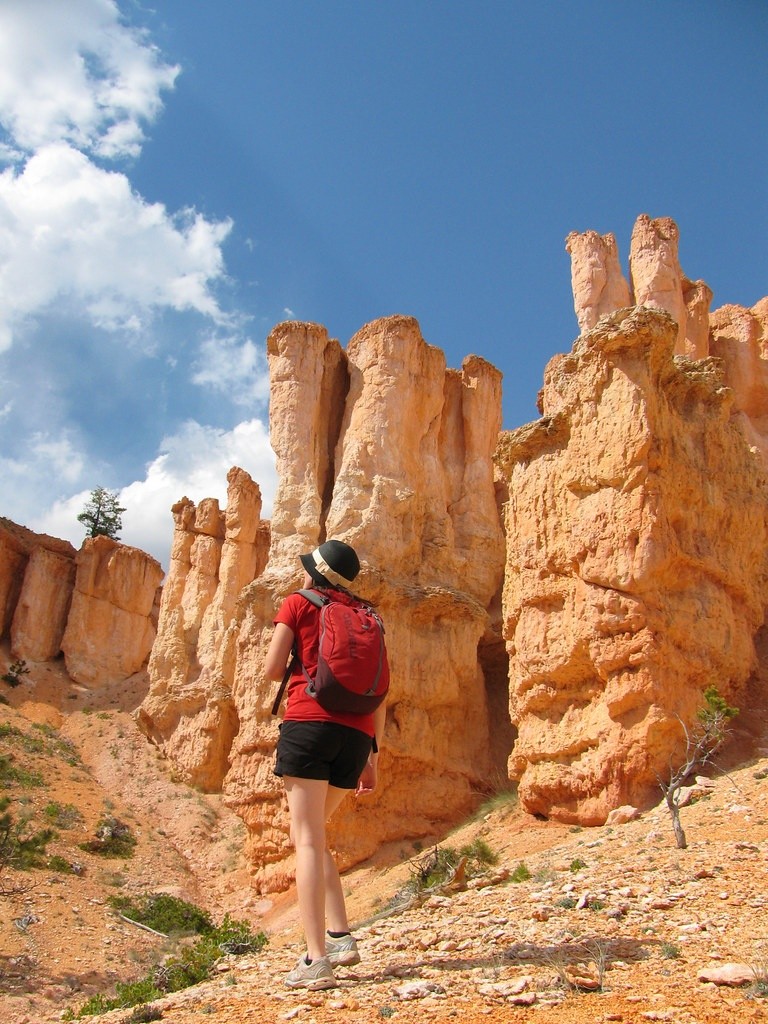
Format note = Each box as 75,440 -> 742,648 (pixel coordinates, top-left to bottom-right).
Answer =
284,957 -> 336,990
325,932 -> 360,969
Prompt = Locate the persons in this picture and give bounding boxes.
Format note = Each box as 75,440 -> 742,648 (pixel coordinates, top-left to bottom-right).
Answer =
263,539 -> 391,992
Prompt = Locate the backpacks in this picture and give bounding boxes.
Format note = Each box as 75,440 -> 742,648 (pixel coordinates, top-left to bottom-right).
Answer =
271,589 -> 390,717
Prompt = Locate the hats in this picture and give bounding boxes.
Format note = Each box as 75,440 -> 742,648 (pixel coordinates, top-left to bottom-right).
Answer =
298,540 -> 360,590
